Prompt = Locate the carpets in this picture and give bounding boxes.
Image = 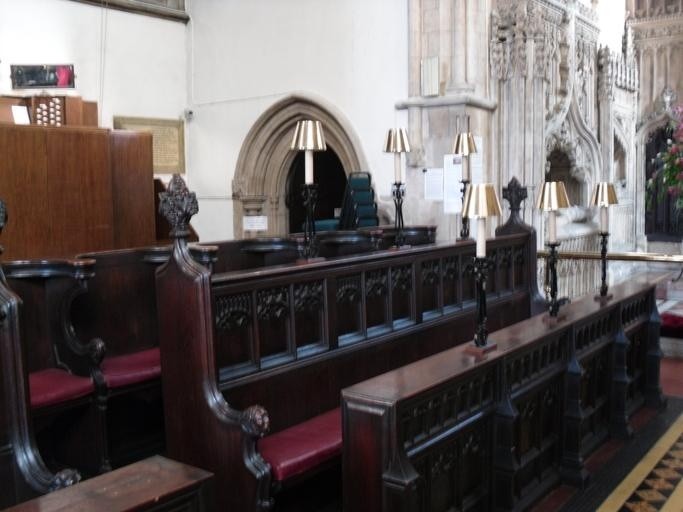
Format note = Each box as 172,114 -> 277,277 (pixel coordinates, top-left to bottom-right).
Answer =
556,390 -> 683,512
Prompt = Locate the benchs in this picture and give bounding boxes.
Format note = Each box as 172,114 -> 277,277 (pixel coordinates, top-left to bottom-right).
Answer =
2,226 -> 345,512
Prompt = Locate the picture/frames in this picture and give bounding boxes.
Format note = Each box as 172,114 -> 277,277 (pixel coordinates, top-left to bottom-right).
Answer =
112,116 -> 185,174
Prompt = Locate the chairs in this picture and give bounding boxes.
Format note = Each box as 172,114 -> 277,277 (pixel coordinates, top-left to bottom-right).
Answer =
302,168 -> 379,234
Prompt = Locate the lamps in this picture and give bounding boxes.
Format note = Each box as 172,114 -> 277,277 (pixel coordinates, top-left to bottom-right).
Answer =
460,184 -> 505,259
539,181 -> 572,243
385,128 -> 410,181
453,131 -> 479,182
291,118 -> 328,185
593,183 -> 619,232
